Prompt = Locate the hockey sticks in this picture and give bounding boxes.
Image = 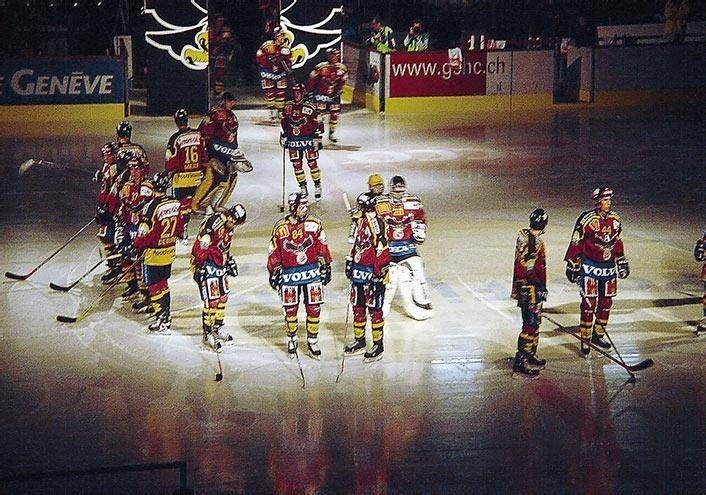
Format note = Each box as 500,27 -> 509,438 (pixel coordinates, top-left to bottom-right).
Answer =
49,252 -> 113,292
204,298 -> 223,381
277,290 -> 307,392
276,145 -> 286,211
4,216 -> 96,281
341,193 -> 355,216
18,157 -> 106,178
56,248 -> 148,324
335,278 -> 353,383
539,312 -> 654,372
580,289 -> 637,383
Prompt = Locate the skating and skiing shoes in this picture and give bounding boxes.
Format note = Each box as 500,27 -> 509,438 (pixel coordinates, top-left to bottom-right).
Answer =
578,335 -> 592,357
328,132 -> 339,146
298,185 -> 308,204
204,330 -> 223,352
595,332 -> 611,352
529,353 -> 541,366
133,300 -> 149,309
215,325 -> 233,341
314,185 -> 323,207
513,359 -> 540,379
308,338 -> 322,358
364,343 -> 383,361
278,112 -> 283,122
344,338 -> 367,355
694,322 -> 705,338
269,110 -> 277,123
101,270 -> 120,286
122,285 -> 140,301
287,332 -> 299,358
145,316 -> 173,336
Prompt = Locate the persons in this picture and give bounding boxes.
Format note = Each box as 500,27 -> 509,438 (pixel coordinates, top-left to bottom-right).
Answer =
102,122 -> 150,177
190,91 -> 252,212
404,19 -> 429,51
281,84 -> 325,203
511,209 -> 548,380
694,234 -> 706,333
347,173 -> 389,246
134,171 -> 183,336
208,13 -> 235,96
565,185 -> 630,359
344,192 -> 391,361
268,194 -> 332,359
113,158 -> 154,314
96,142 -> 126,285
368,174 -> 433,321
365,18 -> 397,53
164,110 -> 210,239
190,205 -> 247,354
308,48 -> 349,143
257,27 -> 292,118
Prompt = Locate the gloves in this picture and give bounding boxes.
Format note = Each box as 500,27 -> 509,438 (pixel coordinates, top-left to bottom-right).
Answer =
411,222 -> 428,244
232,153 -> 254,174
194,267 -> 207,282
319,264 -> 332,285
529,302 -> 544,321
567,263 -> 585,287
226,256 -> 238,277
617,256 -> 631,282
278,131 -> 289,150
313,134 -> 323,154
345,258 -> 354,280
269,269 -> 282,290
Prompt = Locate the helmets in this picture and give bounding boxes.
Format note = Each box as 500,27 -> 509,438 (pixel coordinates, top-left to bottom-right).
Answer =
230,202 -> 248,225
525,206 -> 549,228
173,108 -> 188,120
355,191 -> 376,211
212,107 -> 227,121
326,46 -> 339,56
286,190 -> 308,209
595,186 -> 615,200
291,82 -> 305,91
116,120 -> 132,135
391,176 -> 405,188
274,27 -> 283,35
367,174 -> 384,187
153,171 -> 169,190
98,140 -> 118,154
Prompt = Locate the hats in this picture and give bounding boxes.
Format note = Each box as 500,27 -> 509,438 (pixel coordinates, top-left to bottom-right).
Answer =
222,91 -> 237,103
